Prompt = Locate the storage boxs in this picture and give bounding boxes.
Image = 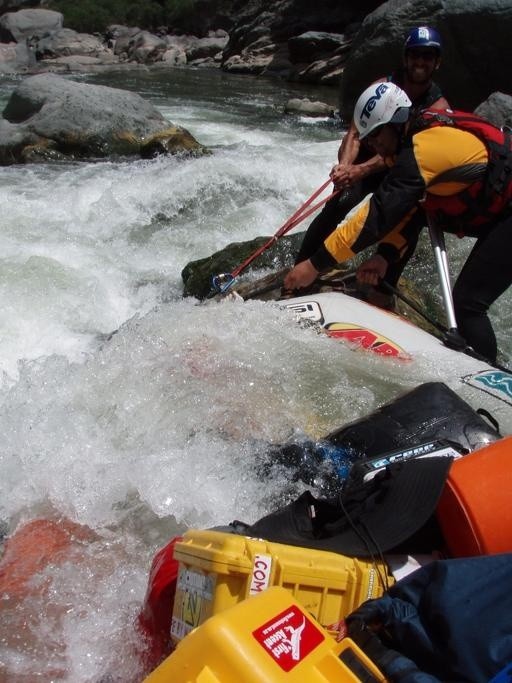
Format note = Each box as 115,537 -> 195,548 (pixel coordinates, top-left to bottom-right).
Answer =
170,526 -> 398,647
140,586 -> 398,683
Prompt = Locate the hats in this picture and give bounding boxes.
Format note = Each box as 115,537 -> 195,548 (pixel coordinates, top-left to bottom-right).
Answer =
248,455 -> 452,557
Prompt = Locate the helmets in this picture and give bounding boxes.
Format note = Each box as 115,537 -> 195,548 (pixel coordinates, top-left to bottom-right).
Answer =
402,25 -> 443,54
353,81 -> 413,140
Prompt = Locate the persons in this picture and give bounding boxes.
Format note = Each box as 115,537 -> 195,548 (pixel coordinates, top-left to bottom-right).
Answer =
285,77 -> 511,365
284,26 -> 450,312
